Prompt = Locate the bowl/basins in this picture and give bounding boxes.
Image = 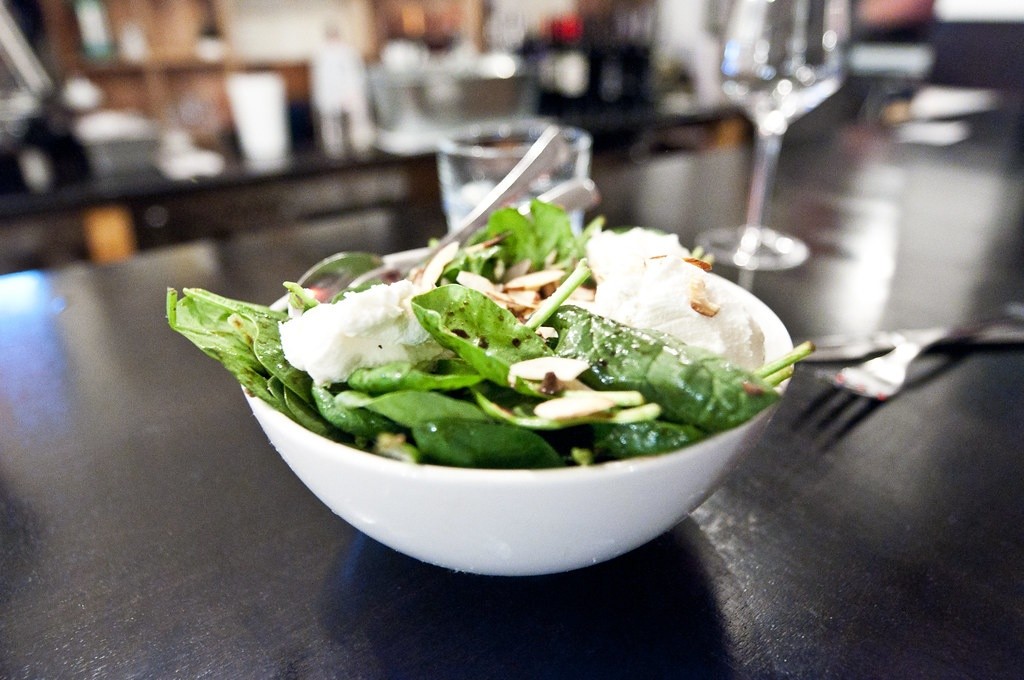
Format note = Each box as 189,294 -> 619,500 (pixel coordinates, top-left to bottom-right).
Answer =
240,249 -> 794,576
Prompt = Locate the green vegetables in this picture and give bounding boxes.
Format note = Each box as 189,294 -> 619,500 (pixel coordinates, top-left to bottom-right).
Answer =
162,195 -> 818,470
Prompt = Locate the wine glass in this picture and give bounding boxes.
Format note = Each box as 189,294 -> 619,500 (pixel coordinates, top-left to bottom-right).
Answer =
692,0 -> 852,272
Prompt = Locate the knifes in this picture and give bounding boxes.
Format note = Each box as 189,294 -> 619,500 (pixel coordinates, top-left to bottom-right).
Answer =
790,327 -> 1023,361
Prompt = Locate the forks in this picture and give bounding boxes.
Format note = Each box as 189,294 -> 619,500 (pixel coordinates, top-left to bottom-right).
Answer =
797,298 -> 1023,402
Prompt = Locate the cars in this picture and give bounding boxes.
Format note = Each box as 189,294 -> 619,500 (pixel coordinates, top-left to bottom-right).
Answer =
515,1 -> 855,155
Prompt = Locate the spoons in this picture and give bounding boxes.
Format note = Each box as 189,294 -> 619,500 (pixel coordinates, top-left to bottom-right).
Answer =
285,125 -> 567,312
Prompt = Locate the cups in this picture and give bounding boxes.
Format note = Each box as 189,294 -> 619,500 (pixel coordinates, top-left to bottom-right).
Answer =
437,119 -> 591,239
226,73 -> 289,165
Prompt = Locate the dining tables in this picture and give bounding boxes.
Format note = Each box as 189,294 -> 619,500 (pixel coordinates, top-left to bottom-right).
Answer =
0,100 -> 1024,679
